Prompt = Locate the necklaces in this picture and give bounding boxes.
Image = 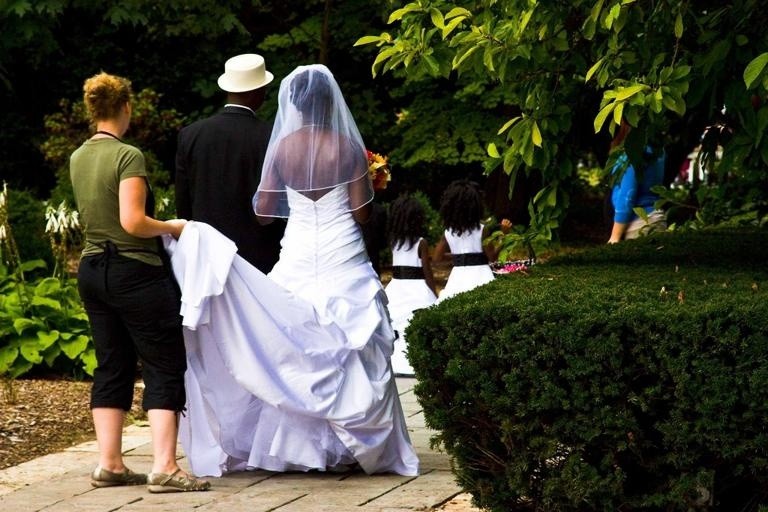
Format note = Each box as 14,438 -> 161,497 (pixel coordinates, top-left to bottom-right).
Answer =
302,124 -> 328,127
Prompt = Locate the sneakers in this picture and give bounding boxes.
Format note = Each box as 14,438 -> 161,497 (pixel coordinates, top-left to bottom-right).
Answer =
148,468 -> 211,493
91,466 -> 147,488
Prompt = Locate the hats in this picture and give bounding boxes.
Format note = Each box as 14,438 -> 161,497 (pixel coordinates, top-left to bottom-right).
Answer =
216,54 -> 275,94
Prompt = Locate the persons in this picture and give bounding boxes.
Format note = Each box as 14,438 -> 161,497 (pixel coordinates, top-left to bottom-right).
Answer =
172,51 -> 286,277
379,192 -> 440,380
250,63 -> 420,482
429,179 -> 513,308
67,67 -> 210,495
602,115 -> 668,247
